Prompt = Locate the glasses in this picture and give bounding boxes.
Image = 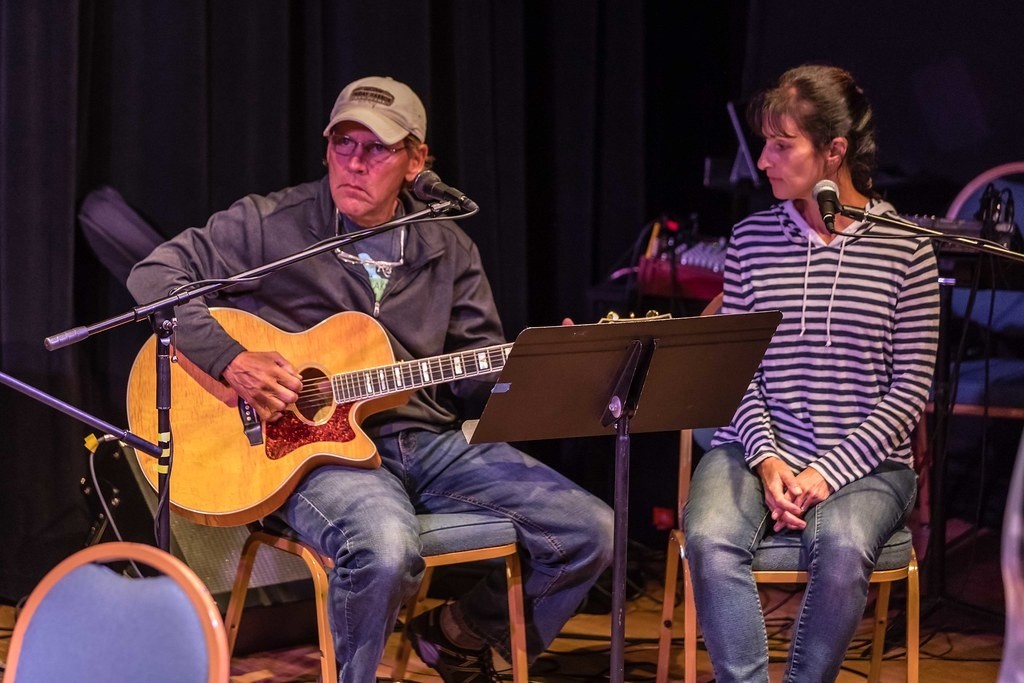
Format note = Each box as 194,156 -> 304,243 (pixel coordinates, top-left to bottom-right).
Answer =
334,207 -> 406,269
331,130 -> 409,162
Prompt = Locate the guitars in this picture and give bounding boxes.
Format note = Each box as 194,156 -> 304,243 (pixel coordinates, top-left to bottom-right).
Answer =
123,305 -> 681,528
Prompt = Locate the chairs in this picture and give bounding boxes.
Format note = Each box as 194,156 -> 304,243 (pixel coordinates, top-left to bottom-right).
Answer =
916,164 -> 1024,562
4,542 -> 230,683
656,411 -> 920,683
224,514 -> 529,683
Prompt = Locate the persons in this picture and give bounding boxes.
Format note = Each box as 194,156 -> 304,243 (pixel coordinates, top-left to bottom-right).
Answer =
127,74 -> 614,683
681,66 -> 941,683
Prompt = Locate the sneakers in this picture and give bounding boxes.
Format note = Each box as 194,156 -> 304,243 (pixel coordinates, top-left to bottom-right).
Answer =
408,604 -> 502,682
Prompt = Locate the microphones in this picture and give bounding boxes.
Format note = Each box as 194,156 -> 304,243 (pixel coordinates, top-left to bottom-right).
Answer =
813,180 -> 839,231
413,170 -> 479,212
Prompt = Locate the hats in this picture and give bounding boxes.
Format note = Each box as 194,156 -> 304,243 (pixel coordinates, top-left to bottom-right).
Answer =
323,76 -> 426,144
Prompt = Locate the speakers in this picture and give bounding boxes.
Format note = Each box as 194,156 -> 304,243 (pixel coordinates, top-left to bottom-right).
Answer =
89,438 -> 321,658
